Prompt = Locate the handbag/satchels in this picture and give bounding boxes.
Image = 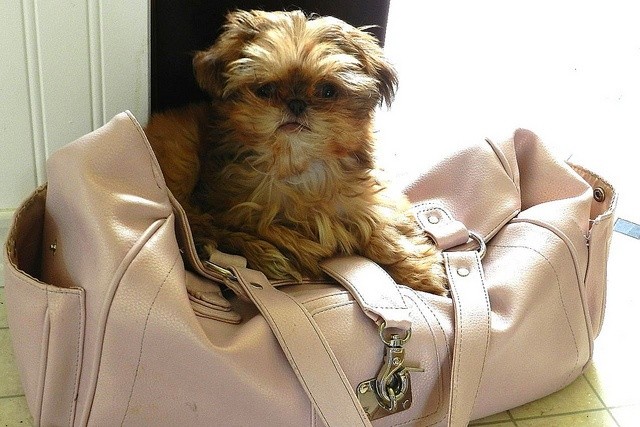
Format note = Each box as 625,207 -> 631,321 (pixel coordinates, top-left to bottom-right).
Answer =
4,110 -> 617,426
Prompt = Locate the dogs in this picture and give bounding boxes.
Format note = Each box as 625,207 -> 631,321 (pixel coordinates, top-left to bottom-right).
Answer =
142,7 -> 452,299
